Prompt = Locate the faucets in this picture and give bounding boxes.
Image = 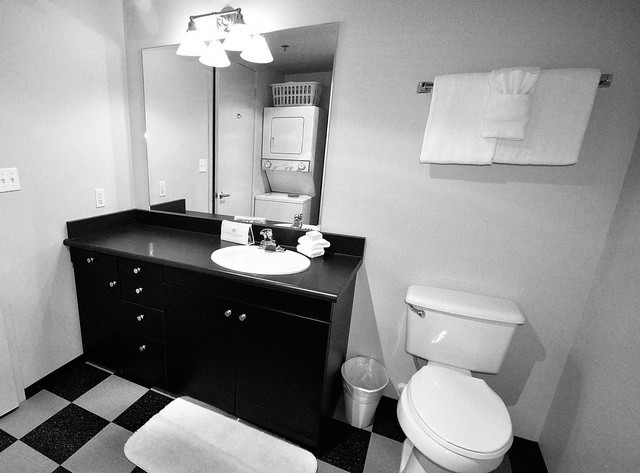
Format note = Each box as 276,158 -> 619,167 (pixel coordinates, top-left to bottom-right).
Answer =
260,226 -> 275,251
293,213 -> 303,230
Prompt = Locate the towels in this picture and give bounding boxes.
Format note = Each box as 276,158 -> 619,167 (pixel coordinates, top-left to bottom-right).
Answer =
296,244 -> 325,258
483,66 -> 540,139
418,70 -> 511,166
298,235 -> 331,249
492,67 -> 602,165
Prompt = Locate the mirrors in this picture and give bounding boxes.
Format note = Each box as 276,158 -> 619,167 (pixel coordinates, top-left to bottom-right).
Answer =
140,21 -> 339,230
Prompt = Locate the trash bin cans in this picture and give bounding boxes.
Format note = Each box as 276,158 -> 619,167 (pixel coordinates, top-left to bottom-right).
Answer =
340,355 -> 391,429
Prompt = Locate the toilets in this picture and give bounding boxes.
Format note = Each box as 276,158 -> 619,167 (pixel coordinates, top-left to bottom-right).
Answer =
397,284 -> 527,473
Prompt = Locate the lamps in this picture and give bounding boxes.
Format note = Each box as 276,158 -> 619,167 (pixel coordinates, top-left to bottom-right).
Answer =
176,0 -> 274,68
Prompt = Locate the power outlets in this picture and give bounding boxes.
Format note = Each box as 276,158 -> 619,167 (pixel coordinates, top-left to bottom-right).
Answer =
96,189 -> 105,208
159,182 -> 165,196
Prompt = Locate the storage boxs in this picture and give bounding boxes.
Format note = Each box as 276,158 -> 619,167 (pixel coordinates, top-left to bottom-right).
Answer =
269,81 -> 324,105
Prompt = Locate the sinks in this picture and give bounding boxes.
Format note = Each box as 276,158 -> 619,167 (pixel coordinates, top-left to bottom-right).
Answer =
210,244 -> 311,274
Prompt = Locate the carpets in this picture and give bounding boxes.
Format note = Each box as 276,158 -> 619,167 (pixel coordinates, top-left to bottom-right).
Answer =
123,396 -> 319,472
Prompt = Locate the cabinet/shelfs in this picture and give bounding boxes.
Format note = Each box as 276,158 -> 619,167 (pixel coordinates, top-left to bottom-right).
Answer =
63,238 -> 170,391
170,257 -> 365,457
